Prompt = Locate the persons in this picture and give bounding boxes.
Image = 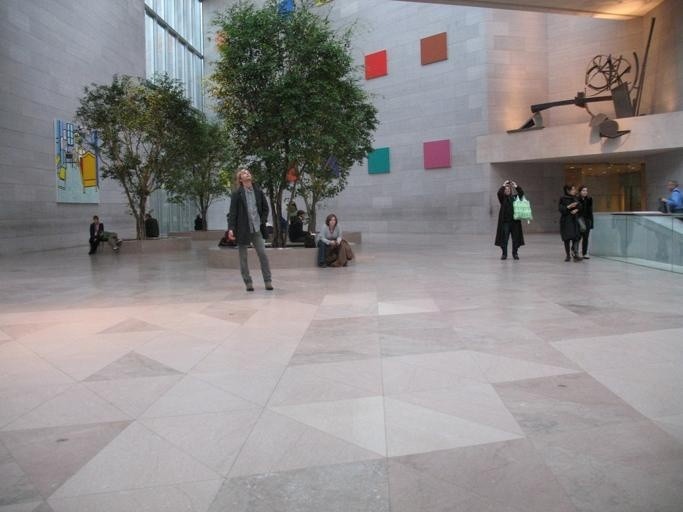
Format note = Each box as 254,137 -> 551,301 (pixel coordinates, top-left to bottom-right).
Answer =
558,183 -> 584,263
193,214 -> 202,230
88,215 -> 104,255
101,231 -> 123,251
494,179 -> 525,260
144,213 -> 160,237
217,168 -> 353,292
660,177 -> 683,213
577,184 -> 594,259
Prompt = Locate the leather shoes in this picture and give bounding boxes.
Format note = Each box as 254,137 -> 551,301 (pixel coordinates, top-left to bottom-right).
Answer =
514,255 -> 519,259
501,255 -> 507,259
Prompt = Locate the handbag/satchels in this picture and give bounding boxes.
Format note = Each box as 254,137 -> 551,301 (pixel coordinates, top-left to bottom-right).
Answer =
513,195 -> 532,220
578,217 -> 587,233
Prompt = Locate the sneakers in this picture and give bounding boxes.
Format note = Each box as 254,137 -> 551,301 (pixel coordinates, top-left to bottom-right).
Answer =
565,253 -> 589,261
247,283 -> 253,291
265,282 -> 273,290
